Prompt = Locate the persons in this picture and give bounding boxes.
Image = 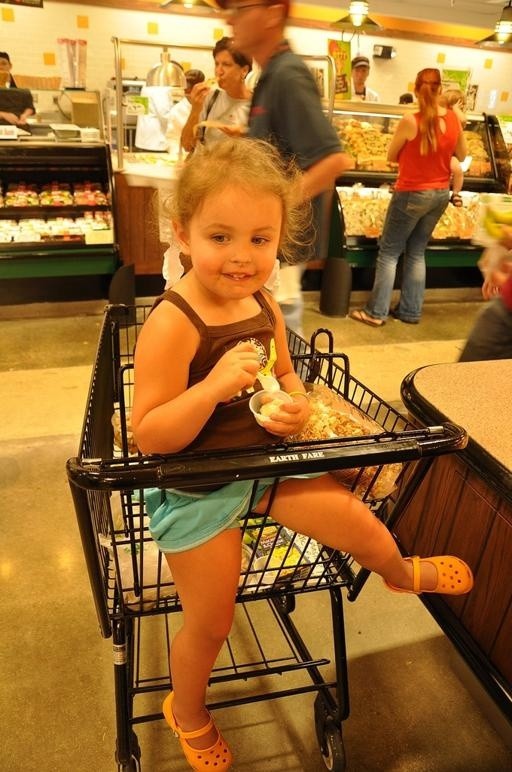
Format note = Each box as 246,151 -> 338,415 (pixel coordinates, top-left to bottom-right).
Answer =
1,52 -> 34,133
132,138 -> 473,771
389,90 -> 466,208
349,68 -> 467,326
457,193 -> 512,362
350,58 -> 382,103
178,37 -> 253,151
216,0 -> 345,383
167,72 -> 206,153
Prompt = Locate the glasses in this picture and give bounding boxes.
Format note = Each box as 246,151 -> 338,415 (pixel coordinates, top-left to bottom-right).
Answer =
227,1 -> 282,19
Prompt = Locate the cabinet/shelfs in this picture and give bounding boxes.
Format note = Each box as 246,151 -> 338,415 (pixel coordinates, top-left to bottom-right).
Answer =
0,143 -> 117,278
321,102 -> 507,276
388,358 -> 512,724
109,152 -> 176,288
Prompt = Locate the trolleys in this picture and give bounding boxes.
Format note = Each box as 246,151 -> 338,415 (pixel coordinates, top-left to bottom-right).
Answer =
63,263 -> 469,772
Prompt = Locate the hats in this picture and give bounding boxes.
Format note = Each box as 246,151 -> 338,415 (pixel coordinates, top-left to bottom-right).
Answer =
352,56 -> 370,69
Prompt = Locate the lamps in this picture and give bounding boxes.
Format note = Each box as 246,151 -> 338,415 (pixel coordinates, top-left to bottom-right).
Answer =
495,0 -> 512,46
347,0 -> 370,27
145,44 -> 188,88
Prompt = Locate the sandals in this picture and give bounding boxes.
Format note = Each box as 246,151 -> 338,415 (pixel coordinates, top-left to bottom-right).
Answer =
384,553 -> 476,597
162,689 -> 233,772
450,193 -> 463,206
350,308 -> 386,330
388,308 -> 421,325
